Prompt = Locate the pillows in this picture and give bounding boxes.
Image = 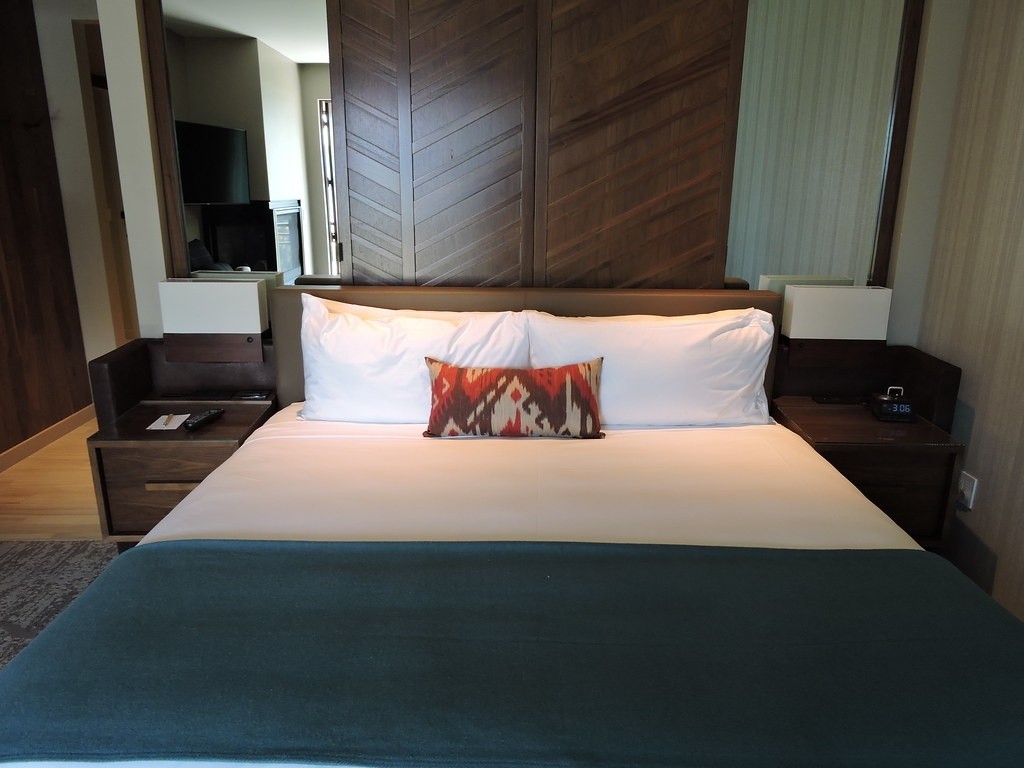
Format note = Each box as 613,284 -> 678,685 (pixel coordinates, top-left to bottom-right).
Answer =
524,306 -> 775,429
296,292 -> 531,426
422,355 -> 608,440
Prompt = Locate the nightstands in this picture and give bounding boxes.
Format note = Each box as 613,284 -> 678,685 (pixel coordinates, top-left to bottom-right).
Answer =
86,336 -> 279,557
767,337 -> 971,557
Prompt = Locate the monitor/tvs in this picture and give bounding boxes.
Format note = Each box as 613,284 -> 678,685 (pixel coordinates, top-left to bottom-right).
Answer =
174,120 -> 251,205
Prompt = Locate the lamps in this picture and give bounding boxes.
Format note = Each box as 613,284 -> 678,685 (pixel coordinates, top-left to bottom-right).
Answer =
779,285 -> 893,367
757,274 -> 856,299
159,275 -> 272,365
189,270 -> 285,338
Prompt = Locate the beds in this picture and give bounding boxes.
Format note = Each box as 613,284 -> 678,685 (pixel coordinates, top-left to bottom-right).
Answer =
0,285 -> 1024,768
